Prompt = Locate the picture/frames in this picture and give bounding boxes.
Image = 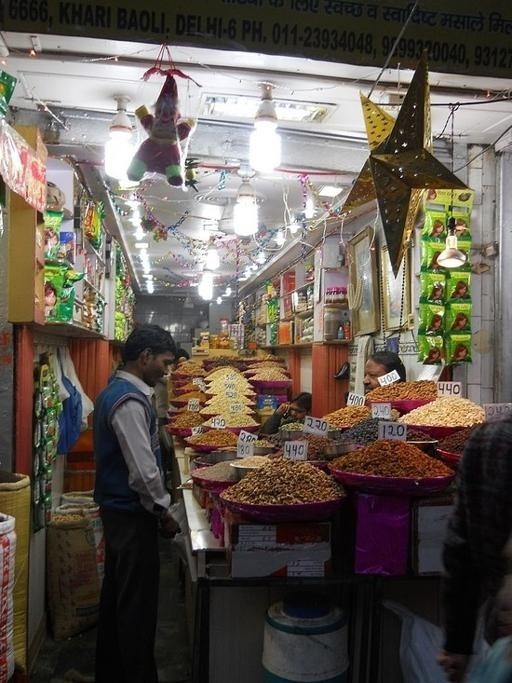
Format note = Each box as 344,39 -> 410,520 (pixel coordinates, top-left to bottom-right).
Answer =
348,224 -> 412,336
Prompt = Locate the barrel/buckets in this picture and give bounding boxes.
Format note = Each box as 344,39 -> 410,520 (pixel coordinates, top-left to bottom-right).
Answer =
261,598 -> 351,683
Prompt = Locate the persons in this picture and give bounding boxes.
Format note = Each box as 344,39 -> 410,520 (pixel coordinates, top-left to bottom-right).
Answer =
257,392 -> 311,441
173,348 -> 190,371
434,410 -> 512,682
43,229 -> 58,252
91,321 -> 182,681
424,189 -> 476,364
44,284 -> 57,315
155,362 -> 169,505
361,349 -> 406,395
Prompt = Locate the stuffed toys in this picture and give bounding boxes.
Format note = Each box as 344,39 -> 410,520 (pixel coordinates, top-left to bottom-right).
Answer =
126,73 -> 195,185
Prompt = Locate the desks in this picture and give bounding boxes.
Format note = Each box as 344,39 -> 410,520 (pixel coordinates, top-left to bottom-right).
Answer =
173,434 -> 365,680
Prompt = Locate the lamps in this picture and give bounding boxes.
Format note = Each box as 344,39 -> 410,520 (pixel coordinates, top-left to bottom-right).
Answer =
436,102 -> 467,268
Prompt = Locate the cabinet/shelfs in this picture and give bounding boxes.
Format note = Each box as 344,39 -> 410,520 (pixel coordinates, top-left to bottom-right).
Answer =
7,156 -> 136,342
234,232 -> 354,347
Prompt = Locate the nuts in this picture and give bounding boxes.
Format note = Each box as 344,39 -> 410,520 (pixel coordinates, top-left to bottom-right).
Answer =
169,353 -> 485,483
219,458 -> 346,506
